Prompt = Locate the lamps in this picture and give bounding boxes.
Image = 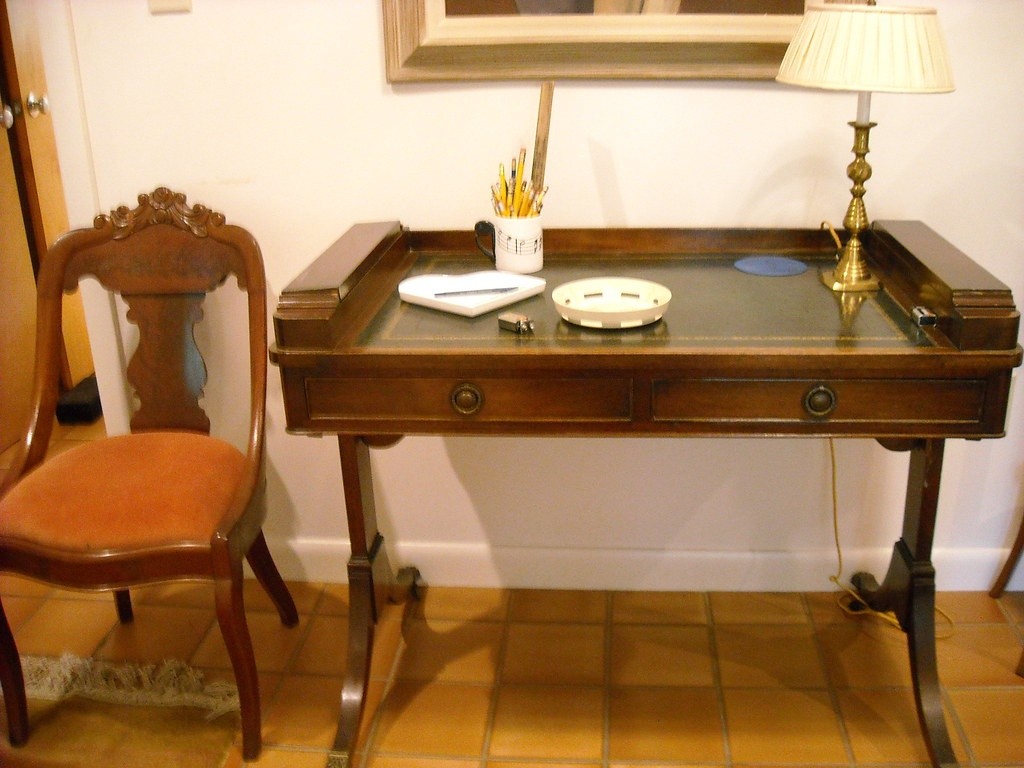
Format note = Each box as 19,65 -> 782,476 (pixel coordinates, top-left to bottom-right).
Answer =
774,2 -> 958,292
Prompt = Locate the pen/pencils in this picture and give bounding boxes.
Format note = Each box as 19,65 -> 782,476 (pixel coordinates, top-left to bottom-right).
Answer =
490,159 -> 548,218
511,148 -> 527,216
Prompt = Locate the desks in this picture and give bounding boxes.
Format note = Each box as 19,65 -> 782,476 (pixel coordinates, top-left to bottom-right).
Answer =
267,219 -> 1023,768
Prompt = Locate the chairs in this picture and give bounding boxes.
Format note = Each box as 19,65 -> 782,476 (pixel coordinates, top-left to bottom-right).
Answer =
0,186 -> 298,757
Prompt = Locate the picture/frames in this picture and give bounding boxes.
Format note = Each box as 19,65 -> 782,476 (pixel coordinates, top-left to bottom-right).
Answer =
380,0 -> 822,85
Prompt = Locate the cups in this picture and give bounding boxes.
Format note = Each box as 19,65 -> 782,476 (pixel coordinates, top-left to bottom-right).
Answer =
474,216 -> 543,275
